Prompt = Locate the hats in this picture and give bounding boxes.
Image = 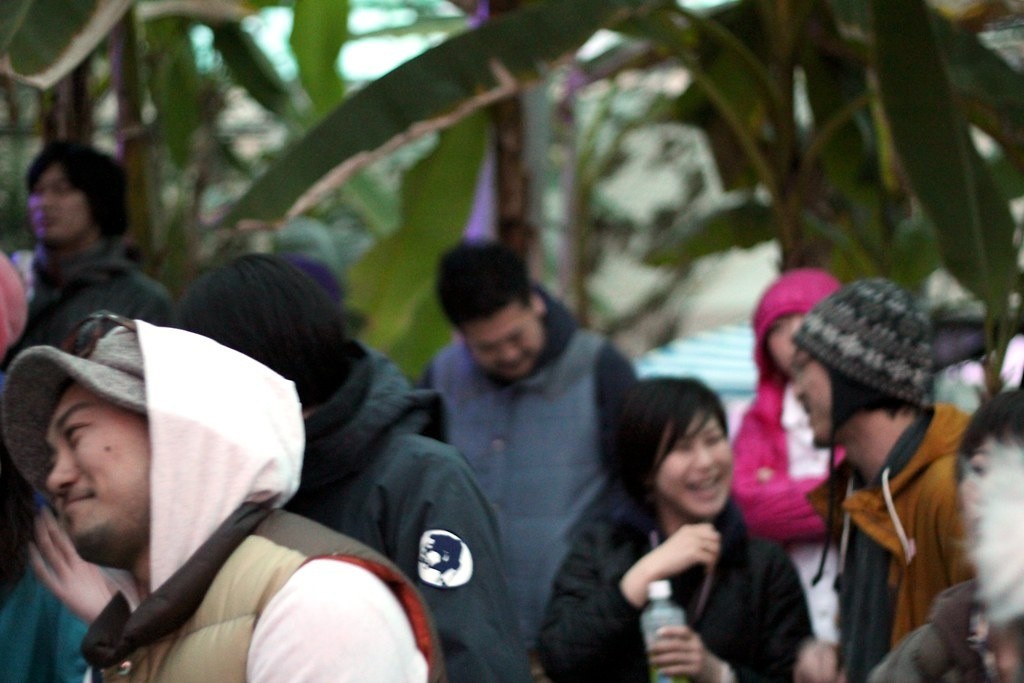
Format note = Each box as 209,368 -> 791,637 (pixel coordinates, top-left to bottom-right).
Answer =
0,325 -> 146,504
791,279 -> 935,410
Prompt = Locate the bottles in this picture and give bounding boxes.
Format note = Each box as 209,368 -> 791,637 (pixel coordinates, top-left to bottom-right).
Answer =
640,579 -> 690,683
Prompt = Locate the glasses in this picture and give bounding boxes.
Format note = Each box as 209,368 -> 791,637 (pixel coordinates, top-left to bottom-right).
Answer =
59,311 -> 136,359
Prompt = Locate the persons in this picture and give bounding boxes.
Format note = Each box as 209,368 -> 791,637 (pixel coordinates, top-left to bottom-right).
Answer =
791,277 -> 977,683
175,252 -> 527,683
1,137 -> 179,683
867,388 -> 1024,683
417,236 -> 639,683
537,378 -> 814,683
0,309 -> 451,683
731,268 -> 848,683
